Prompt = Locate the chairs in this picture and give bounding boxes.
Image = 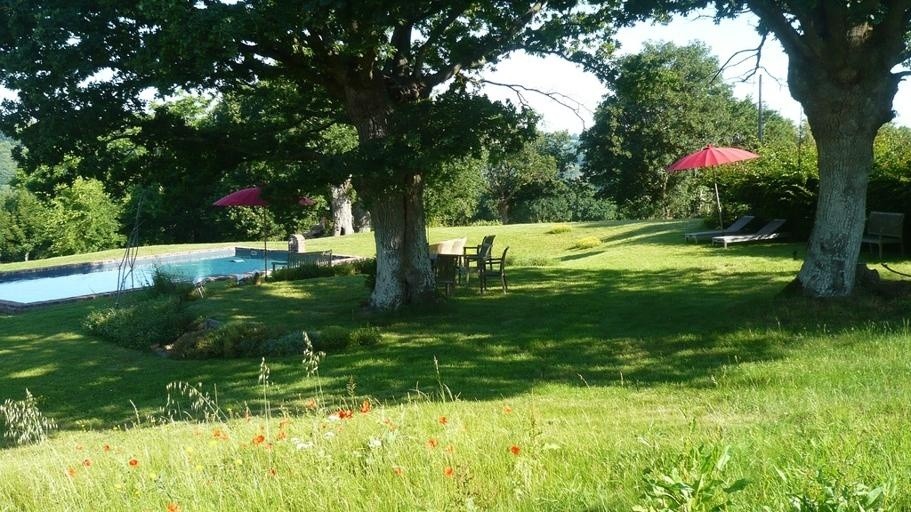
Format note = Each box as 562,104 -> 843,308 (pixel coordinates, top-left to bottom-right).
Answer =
428,234 -> 510,296
684,213 -> 786,249
861,210 -> 905,259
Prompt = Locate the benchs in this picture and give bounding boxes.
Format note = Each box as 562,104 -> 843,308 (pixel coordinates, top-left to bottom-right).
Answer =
271,249 -> 333,271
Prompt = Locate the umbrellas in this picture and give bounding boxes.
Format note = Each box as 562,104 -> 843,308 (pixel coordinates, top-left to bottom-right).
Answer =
665,144 -> 761,231
213,183 -> 317,279
